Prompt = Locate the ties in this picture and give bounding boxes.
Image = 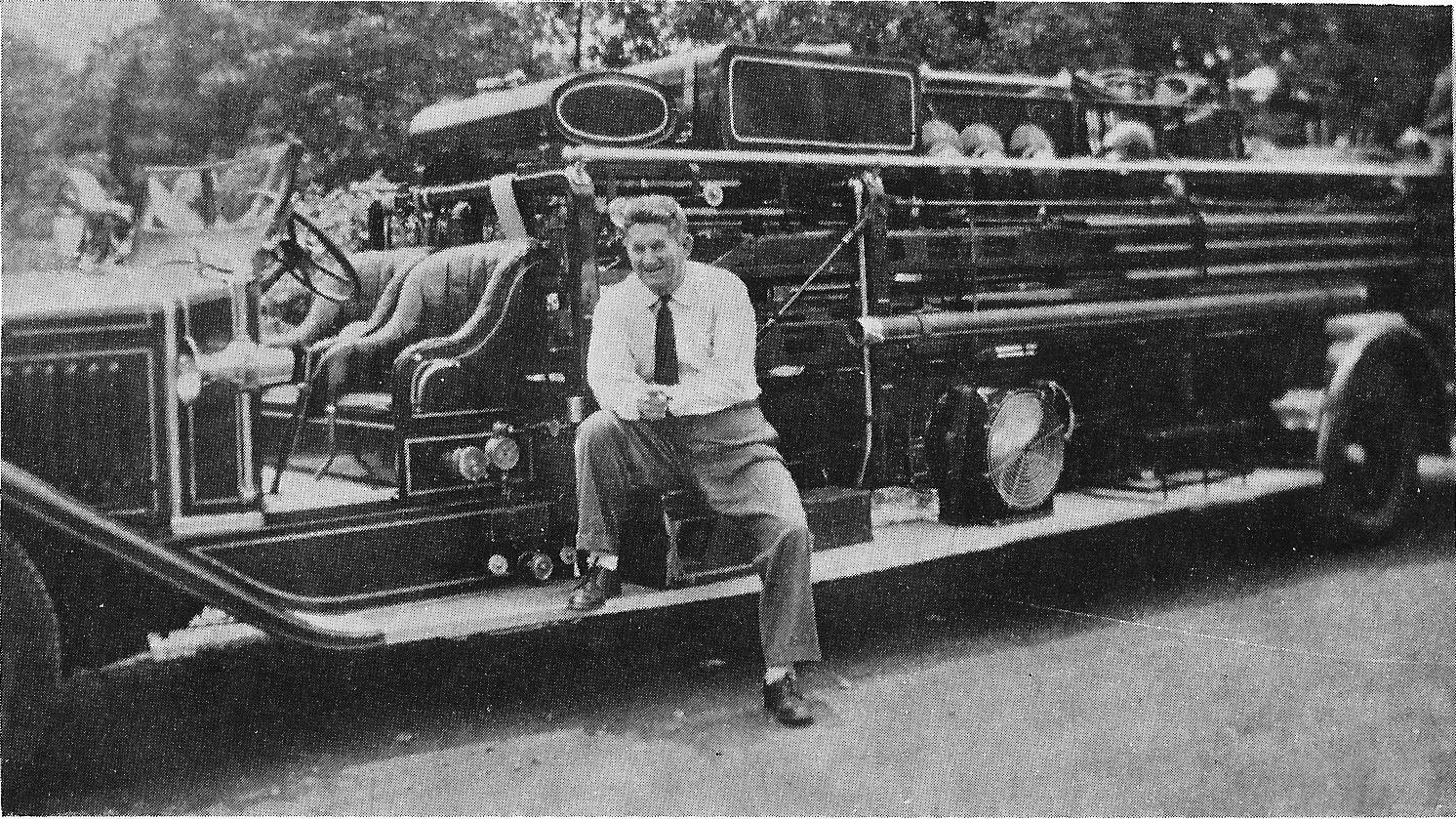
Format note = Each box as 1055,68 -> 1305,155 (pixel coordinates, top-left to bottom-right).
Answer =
654,294 -> 679,386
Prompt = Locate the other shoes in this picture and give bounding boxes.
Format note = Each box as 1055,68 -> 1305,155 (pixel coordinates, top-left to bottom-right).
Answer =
568,556 -> 622,611
762,672 -> 813,726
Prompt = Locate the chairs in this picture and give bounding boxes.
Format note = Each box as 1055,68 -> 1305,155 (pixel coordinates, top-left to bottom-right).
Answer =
252,233 -> 554,489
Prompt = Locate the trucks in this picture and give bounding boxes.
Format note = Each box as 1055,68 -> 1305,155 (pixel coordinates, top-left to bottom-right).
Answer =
0,43 -> 1456,816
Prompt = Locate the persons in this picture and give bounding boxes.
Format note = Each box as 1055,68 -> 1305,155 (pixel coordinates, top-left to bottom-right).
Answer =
567,197 -> 823,730
